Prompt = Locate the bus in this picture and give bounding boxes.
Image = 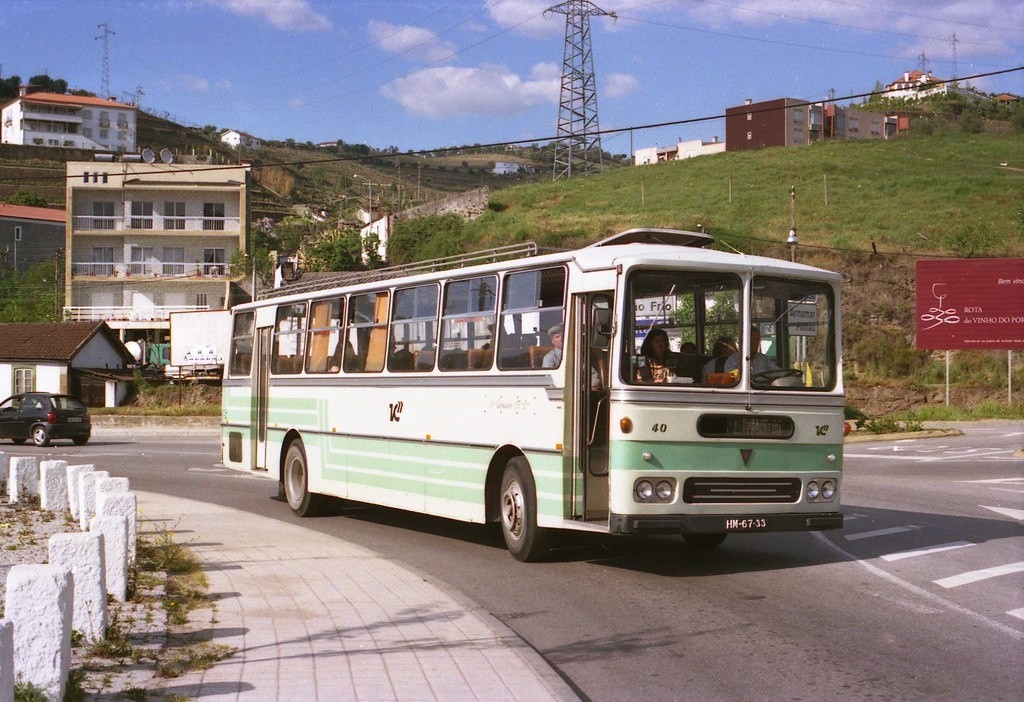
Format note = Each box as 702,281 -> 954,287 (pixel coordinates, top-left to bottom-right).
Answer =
219,223 -> 846,561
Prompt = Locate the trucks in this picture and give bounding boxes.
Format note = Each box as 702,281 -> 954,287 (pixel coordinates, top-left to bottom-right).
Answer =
125,341 -> 171,366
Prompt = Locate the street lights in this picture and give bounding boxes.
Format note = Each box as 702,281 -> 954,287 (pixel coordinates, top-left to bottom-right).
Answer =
354,175 -> 372,233
787,227 -> 797,368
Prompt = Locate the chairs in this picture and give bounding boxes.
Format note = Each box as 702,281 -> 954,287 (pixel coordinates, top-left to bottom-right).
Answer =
23,399 -> 36,412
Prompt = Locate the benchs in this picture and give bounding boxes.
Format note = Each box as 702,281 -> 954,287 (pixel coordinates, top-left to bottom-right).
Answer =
235,346 -> 777,406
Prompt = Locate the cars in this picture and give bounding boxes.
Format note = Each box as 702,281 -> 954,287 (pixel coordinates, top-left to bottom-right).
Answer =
0,392 -> 92,446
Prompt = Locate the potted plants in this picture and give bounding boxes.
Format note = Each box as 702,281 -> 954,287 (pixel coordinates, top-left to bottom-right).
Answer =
193,260 -> 201,277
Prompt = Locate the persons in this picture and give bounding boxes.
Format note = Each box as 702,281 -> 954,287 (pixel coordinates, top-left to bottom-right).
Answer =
330,338 -> 360,373
541,325 -> 606,445
635,328 -> 688,386
700,336 -> 739,382
680,341 -> 698,355
723,325 -> 798,382
393,321 -> 538,372
